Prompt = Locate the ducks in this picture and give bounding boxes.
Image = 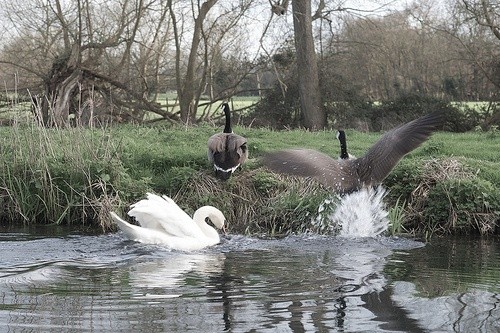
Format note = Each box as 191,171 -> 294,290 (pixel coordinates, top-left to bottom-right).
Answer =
207,102 -> 248,181
258,106 -> 481,197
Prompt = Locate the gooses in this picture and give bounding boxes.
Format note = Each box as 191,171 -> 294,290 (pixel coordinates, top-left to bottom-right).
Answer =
109,191 -> 228,252
